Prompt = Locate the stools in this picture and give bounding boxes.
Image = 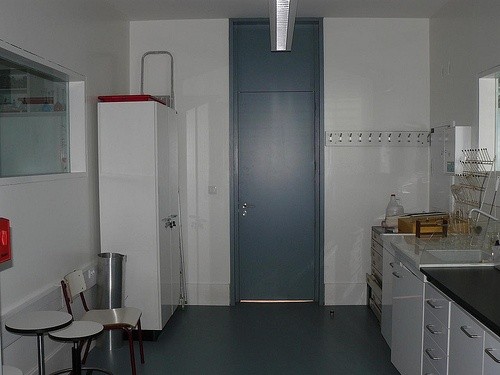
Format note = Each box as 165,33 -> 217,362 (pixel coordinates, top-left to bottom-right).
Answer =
48,321 -> 103,375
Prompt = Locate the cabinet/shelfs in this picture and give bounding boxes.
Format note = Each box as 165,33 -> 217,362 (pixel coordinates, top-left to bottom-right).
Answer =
366,226 -> 500,375
98,102 -> 182,330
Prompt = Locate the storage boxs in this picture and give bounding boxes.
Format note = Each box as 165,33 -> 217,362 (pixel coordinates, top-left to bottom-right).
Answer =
399,217 -> 442,233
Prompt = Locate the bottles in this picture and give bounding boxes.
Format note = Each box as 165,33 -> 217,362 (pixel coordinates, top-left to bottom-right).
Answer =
385,194 -> 404,231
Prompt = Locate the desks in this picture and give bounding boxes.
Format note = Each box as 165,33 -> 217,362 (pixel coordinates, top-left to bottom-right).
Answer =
6,311 -> 73,375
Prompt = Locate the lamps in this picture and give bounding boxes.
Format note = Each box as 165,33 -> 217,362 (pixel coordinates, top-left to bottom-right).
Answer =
269,0 -> 296,52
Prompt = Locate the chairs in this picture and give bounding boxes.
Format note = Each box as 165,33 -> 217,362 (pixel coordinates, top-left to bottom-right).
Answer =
61,269 -> 144,375
